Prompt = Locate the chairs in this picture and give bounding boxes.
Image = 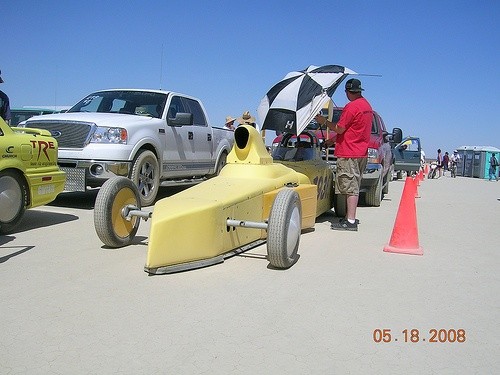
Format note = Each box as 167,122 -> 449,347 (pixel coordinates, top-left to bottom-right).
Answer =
119,99 -> 140,114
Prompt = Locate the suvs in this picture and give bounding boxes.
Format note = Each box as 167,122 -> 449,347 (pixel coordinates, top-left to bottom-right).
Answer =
272,105 -> 423,208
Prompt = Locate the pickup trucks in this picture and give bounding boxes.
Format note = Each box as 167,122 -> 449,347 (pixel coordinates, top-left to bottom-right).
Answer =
15,87 -> 239,208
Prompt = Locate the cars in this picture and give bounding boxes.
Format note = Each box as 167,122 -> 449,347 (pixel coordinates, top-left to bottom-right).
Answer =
0,105 -> 73,236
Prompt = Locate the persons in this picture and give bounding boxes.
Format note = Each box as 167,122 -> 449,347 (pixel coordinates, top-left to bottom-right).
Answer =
223,111 -> 257,129
316,79 -> 373,231
489,153 -> 499,181
0,70 -> 13,119
436,148 -> 460,176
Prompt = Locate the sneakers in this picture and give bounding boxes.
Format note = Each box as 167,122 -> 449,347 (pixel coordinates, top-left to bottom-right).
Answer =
330,218 -> 360,231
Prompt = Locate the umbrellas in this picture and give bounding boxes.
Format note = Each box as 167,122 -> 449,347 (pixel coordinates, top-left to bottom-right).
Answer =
258,64 -> 383,142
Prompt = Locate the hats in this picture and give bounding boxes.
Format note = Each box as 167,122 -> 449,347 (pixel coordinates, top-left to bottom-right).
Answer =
345,78 -> 365,91
236,111 -> 256,125
225,115 -> 236,125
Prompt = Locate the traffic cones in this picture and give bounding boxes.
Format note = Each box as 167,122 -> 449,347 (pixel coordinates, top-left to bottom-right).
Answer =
412,176 -> 422,199
418,163 -> 429,182
383,176 -> 424,257
